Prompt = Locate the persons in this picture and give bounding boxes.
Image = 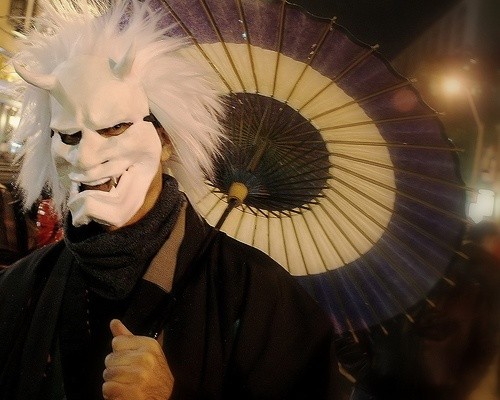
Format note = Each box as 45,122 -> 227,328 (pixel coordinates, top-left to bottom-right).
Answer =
440,219 -> 499,367
0,65 -> 343,399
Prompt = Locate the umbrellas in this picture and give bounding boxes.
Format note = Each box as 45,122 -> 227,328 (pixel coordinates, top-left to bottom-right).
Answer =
111,0 -> 467,344
